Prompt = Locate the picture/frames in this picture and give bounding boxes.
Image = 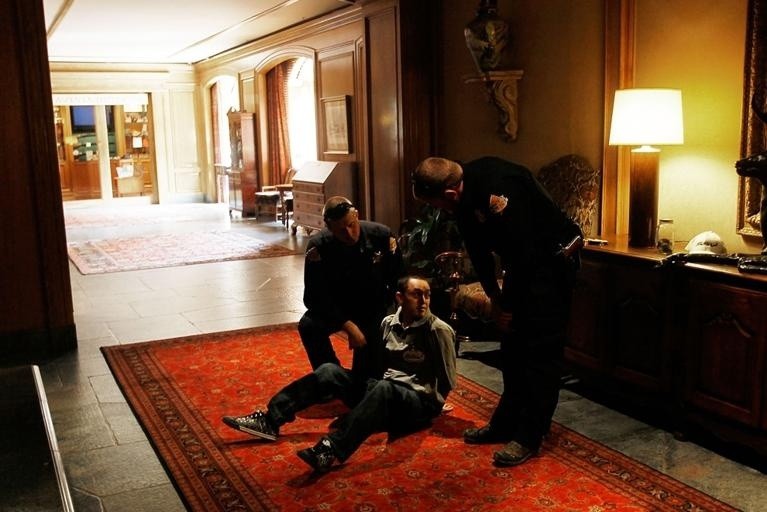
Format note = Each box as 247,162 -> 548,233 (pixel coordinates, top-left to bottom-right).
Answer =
318,94 -> 351,156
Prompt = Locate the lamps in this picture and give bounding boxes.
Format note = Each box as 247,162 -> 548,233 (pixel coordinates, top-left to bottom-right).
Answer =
608,88 -> 686,249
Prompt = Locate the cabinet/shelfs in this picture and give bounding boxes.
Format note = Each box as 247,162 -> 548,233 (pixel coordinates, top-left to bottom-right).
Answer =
225,112 -> 258,218
565,232 -> 765,474
125,112 -> 149,154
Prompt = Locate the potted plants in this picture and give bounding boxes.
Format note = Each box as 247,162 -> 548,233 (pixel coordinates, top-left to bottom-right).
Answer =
396,203 -> 460,322
433,153 -> 602,358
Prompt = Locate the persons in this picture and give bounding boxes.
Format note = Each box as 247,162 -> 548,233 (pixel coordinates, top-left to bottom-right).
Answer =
296,195 -> 400,402
408,156 -> 585,468
220,277 -> 458,473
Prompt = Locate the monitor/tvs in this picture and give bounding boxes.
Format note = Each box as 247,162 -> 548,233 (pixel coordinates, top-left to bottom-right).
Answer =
71,106 -> 114,133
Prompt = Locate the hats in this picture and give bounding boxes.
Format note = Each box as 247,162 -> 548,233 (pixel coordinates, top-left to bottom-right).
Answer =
685,231 -> 727,255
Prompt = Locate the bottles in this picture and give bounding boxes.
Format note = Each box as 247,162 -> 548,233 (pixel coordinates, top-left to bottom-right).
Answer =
126,114 -> 132,123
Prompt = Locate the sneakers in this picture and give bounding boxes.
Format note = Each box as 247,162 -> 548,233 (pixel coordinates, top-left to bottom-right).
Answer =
464,422 -> 508,444
222,409 -> 278,441
297,435 -> 336,470
493,441 -> 532,465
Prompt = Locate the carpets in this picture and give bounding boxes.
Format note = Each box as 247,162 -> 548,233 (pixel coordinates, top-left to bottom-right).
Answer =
66,229 -> 305,275
99,322 -> 743,512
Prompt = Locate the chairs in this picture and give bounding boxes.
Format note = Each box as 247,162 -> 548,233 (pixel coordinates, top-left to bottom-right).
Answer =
254,167 -> 302,232
115,162 -> 145,197
